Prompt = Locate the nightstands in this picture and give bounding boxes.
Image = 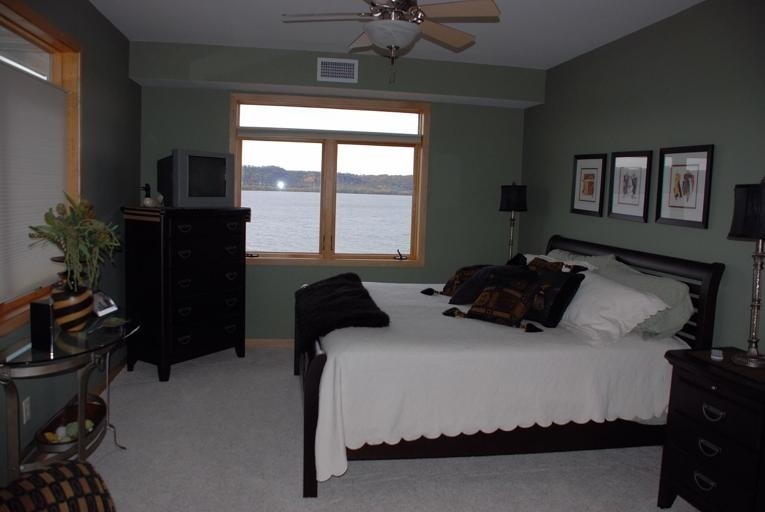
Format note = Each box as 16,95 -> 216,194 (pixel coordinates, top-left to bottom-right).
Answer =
656,346 -> 765,512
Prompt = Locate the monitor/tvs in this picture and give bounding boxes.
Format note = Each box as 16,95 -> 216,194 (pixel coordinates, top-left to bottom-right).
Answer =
157,148 -> 235,209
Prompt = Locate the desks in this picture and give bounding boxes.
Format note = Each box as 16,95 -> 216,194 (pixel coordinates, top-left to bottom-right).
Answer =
124,204 -> 250,382
1,318 -> 126,475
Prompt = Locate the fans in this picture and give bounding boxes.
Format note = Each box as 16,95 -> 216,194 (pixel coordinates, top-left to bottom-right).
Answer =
278,0 -> 501,50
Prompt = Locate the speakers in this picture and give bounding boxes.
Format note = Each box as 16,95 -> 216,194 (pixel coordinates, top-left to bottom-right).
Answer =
29,295 -> 54,353
31,350 -> 53,361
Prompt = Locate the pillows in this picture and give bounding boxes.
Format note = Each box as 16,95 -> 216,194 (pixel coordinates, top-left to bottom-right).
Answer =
441,248 -> 694,337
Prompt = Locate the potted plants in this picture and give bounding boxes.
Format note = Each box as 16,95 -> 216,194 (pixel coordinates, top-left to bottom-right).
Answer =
28,193 -> 122,334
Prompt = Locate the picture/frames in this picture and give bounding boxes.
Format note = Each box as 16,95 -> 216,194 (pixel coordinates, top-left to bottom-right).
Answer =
607,150 -> 652,223
654,144 -> 714,230
569,153 -> 607,217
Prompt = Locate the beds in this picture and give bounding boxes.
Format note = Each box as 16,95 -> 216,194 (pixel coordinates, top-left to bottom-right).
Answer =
296,234 -> 725,497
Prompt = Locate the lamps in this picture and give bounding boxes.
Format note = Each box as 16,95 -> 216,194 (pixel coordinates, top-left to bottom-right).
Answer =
362,20 -> 422,57
725,184 -> 764,367
498,182 -> 527,259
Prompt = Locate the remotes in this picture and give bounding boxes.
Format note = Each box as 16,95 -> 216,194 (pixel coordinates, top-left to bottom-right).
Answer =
711,349 -> 724,362
